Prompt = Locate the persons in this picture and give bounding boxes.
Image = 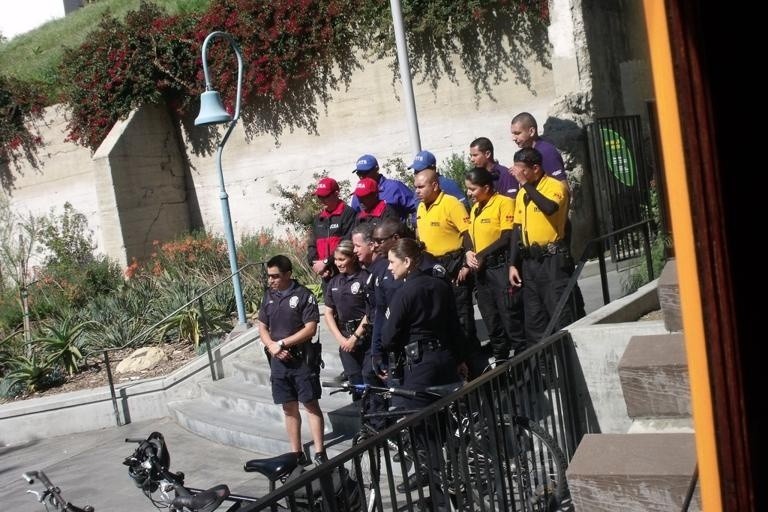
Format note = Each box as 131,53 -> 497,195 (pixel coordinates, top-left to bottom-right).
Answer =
380,238 -> 461,492
507,146 -> 571,394
457,167 -> 528,390
512,111 -> 585,320
305,151 -> 511,465
258,254 -> 333,471
470,137 -> 517,200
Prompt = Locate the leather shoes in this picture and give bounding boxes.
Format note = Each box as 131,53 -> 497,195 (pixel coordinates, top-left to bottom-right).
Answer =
398,469 -> 431,490
394,442 -> 412,461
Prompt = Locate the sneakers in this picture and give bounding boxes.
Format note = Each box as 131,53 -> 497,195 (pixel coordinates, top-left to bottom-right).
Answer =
314,453 -> 325,467
297,452 -> 307,467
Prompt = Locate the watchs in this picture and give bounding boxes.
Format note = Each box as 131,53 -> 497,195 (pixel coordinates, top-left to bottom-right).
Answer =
278,340 -> 286,347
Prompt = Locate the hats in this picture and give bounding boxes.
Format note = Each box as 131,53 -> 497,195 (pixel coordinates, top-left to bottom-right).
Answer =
352,155 -> 377,173
312,178 -> 339,197
408,151 -> 435,171
350,177 -> 378,197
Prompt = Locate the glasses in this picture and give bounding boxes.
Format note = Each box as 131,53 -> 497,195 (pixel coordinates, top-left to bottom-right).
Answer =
267,273 -> 280,279
372,234 -> 393,244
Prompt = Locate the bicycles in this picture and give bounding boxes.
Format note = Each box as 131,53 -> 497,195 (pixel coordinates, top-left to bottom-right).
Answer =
122,430 -> 366,512
322,378 -> 567,512
21,470 -> 96,512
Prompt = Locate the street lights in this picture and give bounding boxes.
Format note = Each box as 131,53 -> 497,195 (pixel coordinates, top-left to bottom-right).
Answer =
195,30 -> 247,327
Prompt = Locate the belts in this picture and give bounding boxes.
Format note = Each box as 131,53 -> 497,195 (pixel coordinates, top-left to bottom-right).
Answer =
420,339 -> 445,350
